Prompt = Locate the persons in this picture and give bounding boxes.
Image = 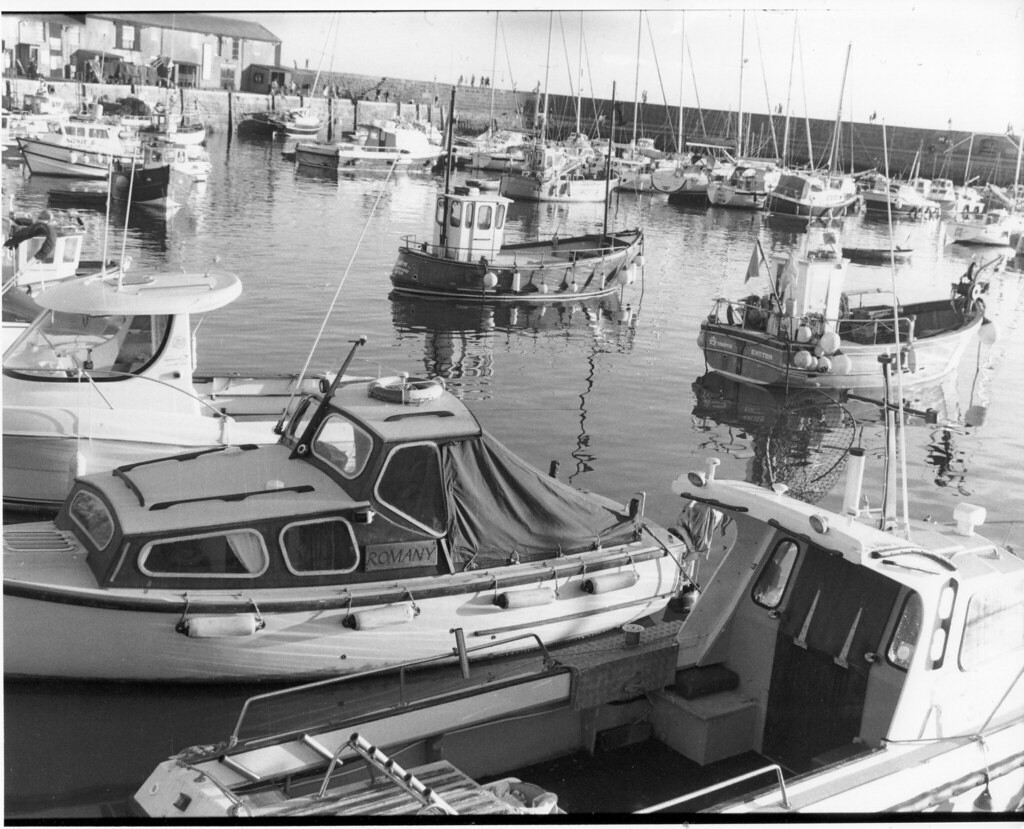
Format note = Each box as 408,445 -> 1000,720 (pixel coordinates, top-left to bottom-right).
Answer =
28,58 -> 35,79
870,112 -> 877,123
268,80 -> 389,102
598,114 -> 606,127
777,103 -> 783,116
458,74 -> 490,88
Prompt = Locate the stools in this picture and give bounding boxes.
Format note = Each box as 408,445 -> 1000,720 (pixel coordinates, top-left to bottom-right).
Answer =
649,683 -> 759,766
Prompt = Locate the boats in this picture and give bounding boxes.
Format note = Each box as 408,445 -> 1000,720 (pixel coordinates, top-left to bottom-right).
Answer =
2,158 -> 402,516
389,85 -> 646,308
695,198 -> 1017,389
240,11 -> 1024,245
129,118 -> 1024,817
1,13 -> 211,221
3,334 -> 722,682
